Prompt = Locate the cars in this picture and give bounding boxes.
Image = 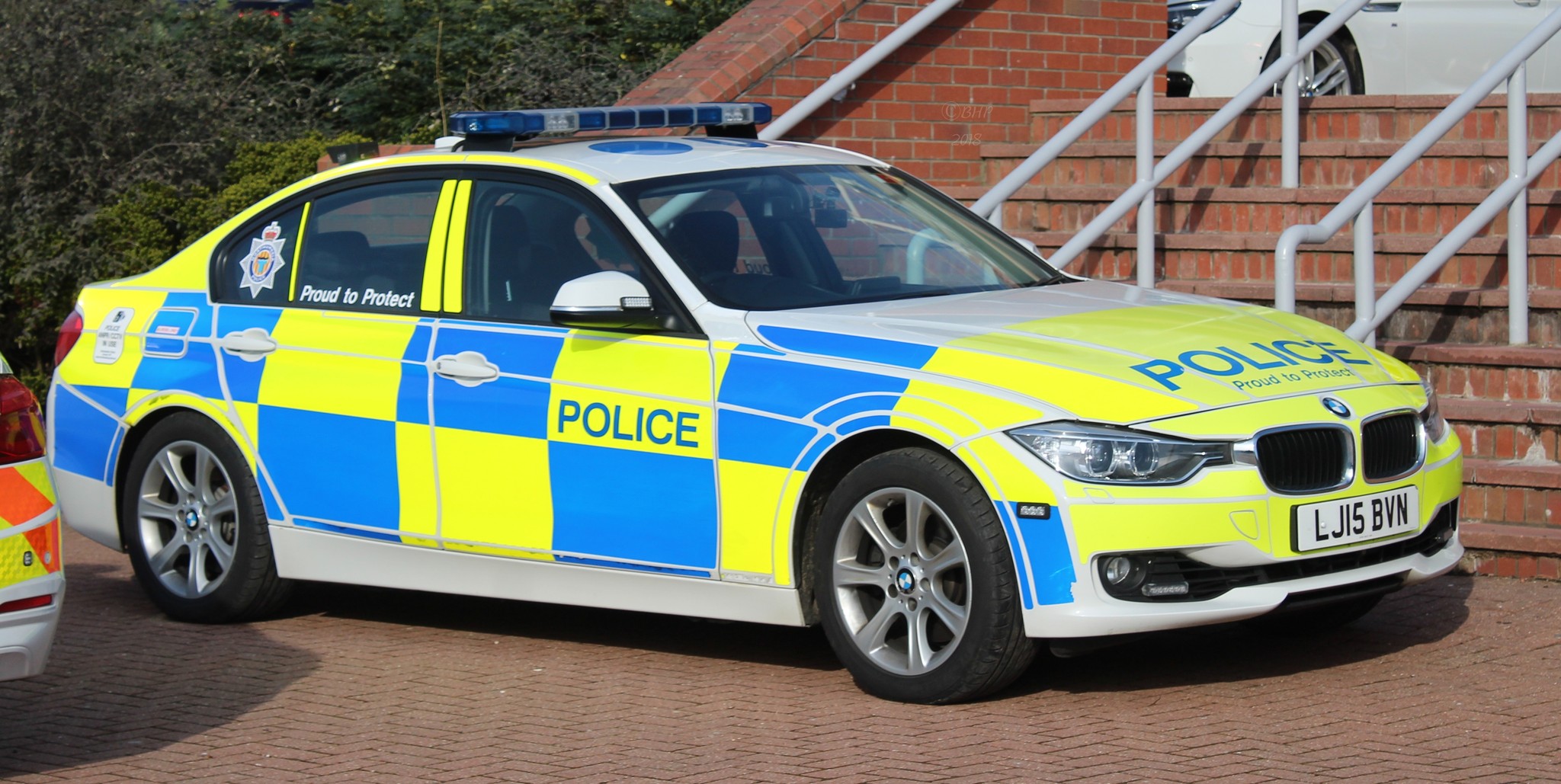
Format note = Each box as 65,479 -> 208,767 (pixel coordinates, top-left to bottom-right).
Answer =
46,101 -> 1463,710
1161,1 -> 1559,97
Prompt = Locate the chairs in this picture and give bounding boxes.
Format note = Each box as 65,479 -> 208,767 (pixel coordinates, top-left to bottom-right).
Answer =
664,209 -> 793,315
